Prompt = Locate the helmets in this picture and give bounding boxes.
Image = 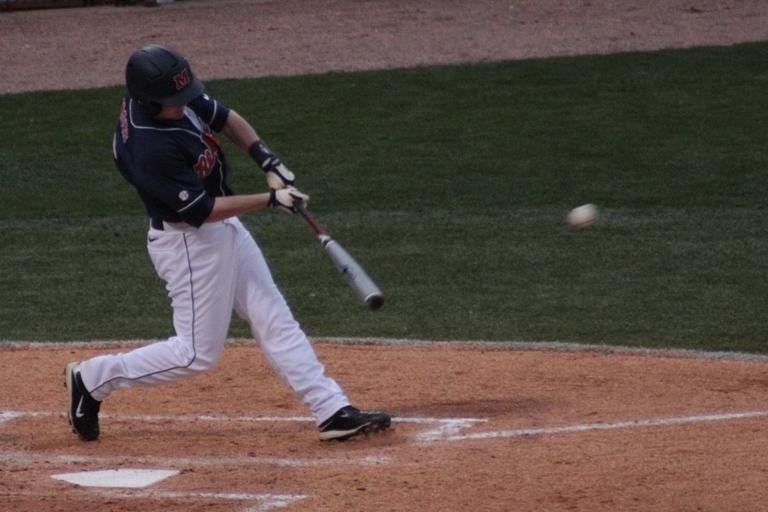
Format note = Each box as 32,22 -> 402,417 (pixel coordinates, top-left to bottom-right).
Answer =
126,45 -> 205,106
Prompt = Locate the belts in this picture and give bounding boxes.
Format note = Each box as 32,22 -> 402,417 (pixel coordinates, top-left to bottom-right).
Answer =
152,219 -> 164,231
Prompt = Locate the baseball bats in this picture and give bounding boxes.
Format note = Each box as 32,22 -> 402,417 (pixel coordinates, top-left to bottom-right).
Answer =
299,208 -> 385,313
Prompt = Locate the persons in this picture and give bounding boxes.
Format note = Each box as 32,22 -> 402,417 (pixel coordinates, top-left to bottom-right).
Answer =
65,46 -> 390,443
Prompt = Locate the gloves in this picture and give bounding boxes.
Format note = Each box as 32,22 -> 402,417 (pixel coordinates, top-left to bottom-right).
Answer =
261,156 -> 310,215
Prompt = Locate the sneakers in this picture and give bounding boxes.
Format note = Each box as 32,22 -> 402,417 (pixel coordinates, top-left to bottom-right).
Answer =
319,405 -> 391,440
64,362 -> 102,442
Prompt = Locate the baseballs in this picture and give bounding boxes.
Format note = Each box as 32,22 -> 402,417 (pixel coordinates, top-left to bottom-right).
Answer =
567,204 -> 597,227
